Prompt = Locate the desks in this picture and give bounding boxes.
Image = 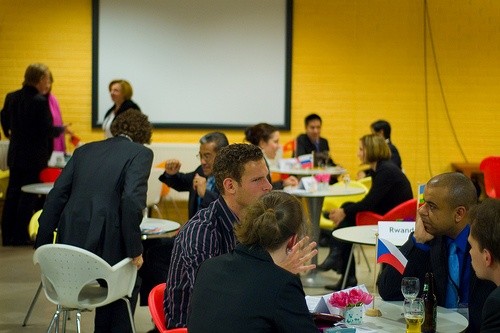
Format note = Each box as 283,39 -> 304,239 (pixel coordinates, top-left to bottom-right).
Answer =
353,296 -> 468,333
139,218 -> 181,237
332,224 -> 379,290
268,165 -> 343,174
21,182 -> 54,193
283,185 -> 367,287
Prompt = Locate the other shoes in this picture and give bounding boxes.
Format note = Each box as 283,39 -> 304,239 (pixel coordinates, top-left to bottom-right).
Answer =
2,239 -> 35,247
325,274 -> 357,292
316,253 -> 345,275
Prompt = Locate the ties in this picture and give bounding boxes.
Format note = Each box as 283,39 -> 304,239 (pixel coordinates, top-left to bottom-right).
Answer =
444,241 -> 460,308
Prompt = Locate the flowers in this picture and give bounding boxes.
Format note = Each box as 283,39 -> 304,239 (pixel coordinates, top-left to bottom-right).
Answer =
328,287 -> 374,308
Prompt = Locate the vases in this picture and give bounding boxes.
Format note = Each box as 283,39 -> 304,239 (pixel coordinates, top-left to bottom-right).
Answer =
341,304 -> 362,325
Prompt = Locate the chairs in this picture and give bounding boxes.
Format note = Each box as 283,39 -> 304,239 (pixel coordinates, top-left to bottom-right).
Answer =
22,144 -> 500,333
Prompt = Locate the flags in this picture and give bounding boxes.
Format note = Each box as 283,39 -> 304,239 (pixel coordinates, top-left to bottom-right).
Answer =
377,238 -> 408,274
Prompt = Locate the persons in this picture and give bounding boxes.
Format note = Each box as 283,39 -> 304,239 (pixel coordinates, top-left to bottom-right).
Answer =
158,132 -> 228,220
316,134 -> 414,291
163,143 -> 323,333
34,109 -> 154,333
102,80 -> 139,137
459,196 -> 500,333
355,119 -> 402,179
245,123 -> 299,190
0,62 -> 73,246
292,113 -> 342,185
377,173 -> 477,333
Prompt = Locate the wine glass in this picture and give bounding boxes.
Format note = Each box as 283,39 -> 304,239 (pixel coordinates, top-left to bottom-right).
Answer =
400,277 -> 420,318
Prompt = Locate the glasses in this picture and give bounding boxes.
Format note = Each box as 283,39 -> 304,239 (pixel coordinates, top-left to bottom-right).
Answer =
194,153 -> 214,161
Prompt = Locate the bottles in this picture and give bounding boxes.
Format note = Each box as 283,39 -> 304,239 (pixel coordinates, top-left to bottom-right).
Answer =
420,280 -> 437,333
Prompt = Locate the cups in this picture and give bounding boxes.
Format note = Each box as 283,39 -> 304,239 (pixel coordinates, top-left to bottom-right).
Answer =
403,298 -> 425,333
317,151 -> 327,170
